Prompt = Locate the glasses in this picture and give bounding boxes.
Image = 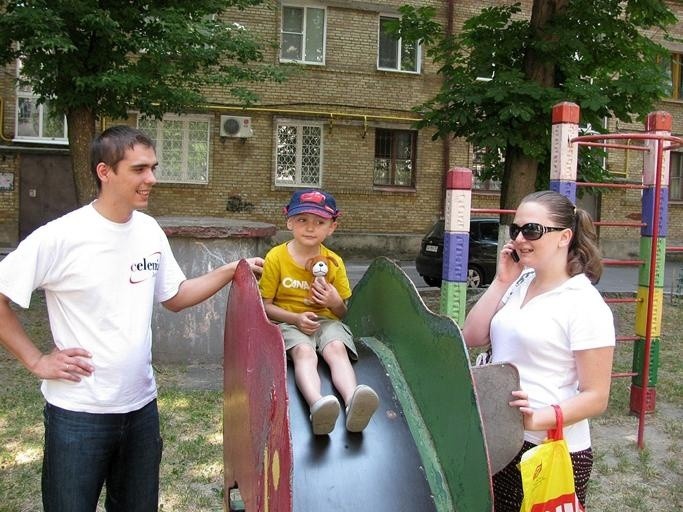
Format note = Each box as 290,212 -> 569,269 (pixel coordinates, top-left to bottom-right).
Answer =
505,216 -> 569,246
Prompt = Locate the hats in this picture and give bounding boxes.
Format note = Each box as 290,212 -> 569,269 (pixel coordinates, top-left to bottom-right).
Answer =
282,187 -> 343,225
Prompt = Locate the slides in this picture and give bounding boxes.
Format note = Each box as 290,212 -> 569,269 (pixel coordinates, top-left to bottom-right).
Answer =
225,256 -> 495,512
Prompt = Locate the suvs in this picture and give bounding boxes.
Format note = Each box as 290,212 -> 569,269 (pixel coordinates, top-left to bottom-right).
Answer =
413,214 -> 499,291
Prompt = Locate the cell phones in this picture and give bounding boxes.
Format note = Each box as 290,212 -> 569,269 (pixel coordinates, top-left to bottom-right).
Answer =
511,248 -> 519,263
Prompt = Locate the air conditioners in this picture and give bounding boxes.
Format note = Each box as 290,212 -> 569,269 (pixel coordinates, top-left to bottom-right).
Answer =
220,114 -> 252,138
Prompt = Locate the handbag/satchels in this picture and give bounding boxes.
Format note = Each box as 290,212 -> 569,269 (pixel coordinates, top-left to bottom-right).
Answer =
514,403 -> 588,512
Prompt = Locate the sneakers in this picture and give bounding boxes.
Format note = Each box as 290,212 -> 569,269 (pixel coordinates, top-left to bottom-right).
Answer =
307,393 -> 341,437
345,382 -> 380,433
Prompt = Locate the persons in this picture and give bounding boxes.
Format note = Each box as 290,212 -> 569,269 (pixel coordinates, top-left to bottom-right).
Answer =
303,256 -> 339,306
463,190 -> 616,512
258,189 -> 379,435
0,125 -> 264,512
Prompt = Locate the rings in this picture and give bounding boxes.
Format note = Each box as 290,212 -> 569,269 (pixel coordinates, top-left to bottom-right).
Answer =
65,365 -> 69,372
